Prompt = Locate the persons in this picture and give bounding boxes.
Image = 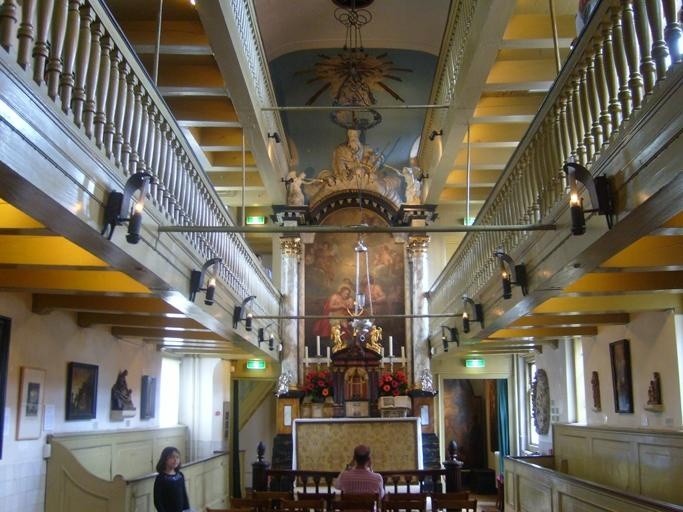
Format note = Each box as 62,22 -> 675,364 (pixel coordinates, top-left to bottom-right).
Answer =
396,167 -> 414,201
293,173 -> 312,205
153,446 -> 190,512
328,284 -> 353,326
335,128 -> 374,180
361,275 -> 386,314
333,445 -> 385,512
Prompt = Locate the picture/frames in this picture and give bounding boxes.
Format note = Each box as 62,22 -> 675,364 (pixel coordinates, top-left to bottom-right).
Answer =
609,338 -> 633,414
141,375 -> 156,420
530,368 -> 549,435
16,365 -> 47,441
65,361 -> 99,421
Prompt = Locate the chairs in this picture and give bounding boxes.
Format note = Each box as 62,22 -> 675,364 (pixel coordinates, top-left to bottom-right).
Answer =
206,488 -> 478,512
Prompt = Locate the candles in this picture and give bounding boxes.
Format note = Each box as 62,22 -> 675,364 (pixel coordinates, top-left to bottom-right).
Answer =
380,335 -> 405,368
304,335 -> 331,368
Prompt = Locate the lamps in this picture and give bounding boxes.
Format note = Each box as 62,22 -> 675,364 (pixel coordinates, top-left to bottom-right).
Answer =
461,296 -> 483,334
440,325 -> 459,352
428,128 -> 442,141
493,252 -> 528,300
233,295 -> 257,332
416,173 -> 429,182
559,156 -> 613,237
258,328 -> 274,351
268,132 -> 281,143
102,169 -> 154,245
281,177 -> 294,185
189,257 -> 222,307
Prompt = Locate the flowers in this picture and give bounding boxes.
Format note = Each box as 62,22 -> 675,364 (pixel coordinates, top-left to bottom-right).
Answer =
299,369 -> 336,403
377,367 -> 409,397
